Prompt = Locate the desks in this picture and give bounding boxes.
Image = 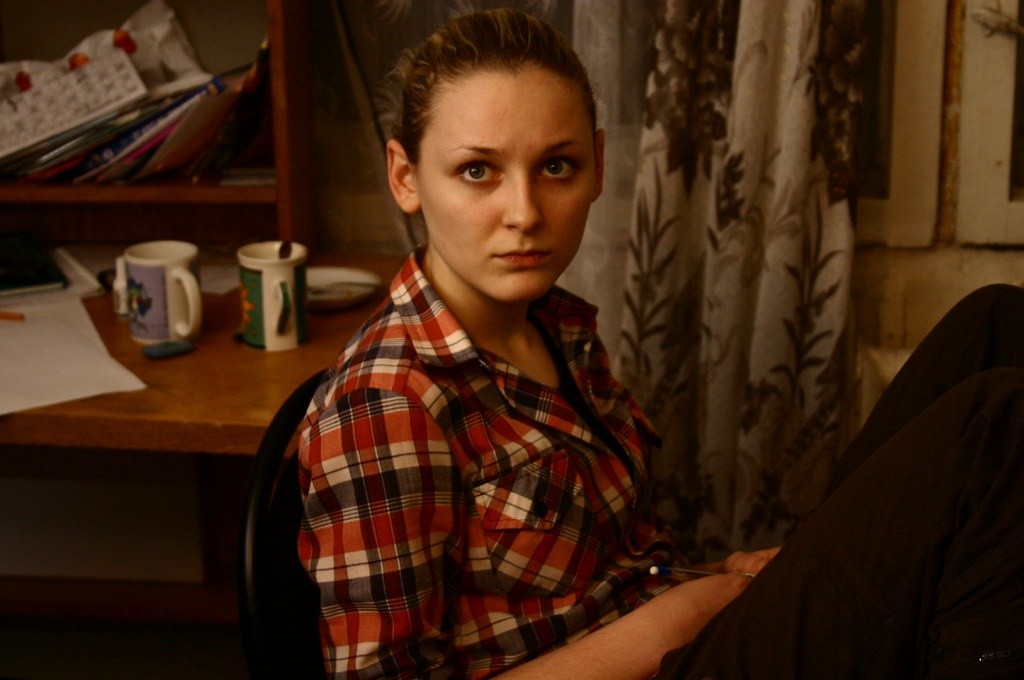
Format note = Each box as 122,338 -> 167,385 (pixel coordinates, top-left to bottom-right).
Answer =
0,245 -> 409,680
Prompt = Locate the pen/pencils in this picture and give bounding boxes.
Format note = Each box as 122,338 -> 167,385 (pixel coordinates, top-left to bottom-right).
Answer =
650,564 -> 721,578
0,310 -> 26,322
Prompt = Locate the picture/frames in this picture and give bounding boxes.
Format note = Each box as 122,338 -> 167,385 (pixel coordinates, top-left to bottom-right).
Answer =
954,0 -> 1024,244
852,0 -> 949,251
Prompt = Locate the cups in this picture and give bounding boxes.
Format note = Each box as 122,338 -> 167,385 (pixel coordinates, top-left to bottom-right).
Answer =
237,241 -> 307,352
124,241 -> 204,346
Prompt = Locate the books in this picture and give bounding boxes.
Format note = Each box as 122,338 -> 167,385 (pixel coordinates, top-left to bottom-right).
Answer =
0,0 -> 279,187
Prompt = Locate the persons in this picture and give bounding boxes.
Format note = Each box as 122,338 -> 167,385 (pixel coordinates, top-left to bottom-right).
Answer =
296,7 -> 1024,680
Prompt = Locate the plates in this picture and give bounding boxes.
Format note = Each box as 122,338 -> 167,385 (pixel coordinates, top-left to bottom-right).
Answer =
305,266 -> 382,310
